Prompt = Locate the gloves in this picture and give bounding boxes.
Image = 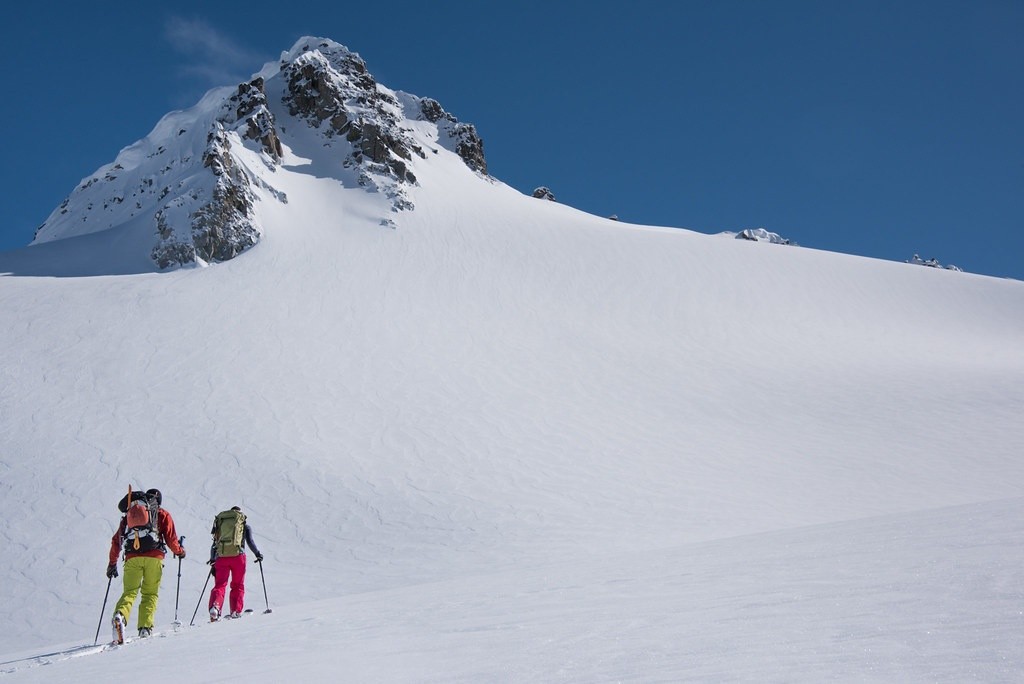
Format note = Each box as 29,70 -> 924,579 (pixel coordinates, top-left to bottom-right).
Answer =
106,564 -> 118,578
256,552 -> 263,561
178,547 -> 186,558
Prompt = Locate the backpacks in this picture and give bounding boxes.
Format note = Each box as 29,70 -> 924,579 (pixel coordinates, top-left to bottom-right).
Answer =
118,491 -> 167,554
211,510 -> 247,558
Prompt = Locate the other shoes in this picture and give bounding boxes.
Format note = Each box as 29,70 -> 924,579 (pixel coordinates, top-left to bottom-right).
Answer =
231,611 -> 241,619
112,613 -> 124,645
139,628 -> 150,638
210,607 -> 218,622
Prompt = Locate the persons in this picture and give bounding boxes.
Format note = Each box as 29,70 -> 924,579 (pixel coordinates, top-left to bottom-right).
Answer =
107,489 -> 185,644
210,506 -> 263,621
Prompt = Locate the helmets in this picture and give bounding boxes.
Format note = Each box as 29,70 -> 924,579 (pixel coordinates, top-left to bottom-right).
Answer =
146,489 -> 162,505
